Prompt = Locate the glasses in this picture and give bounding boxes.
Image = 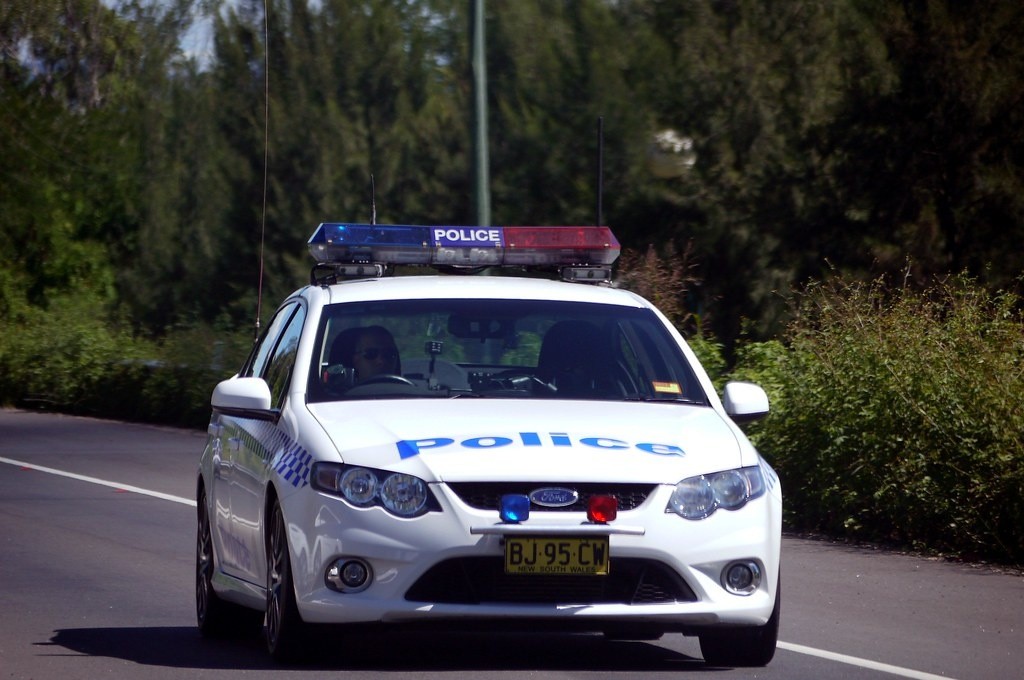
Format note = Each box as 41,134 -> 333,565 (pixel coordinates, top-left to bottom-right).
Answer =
350,347 -> 397,361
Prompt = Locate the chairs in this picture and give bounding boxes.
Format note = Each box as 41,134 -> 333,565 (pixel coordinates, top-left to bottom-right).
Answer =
327,325 -> 401,384
528,319 -> 625,400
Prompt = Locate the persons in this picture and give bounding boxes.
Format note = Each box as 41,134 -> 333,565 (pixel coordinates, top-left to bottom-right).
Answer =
352,326 -> 398,385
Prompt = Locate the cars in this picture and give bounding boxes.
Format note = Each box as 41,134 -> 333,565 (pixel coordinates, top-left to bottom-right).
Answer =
197,213 -> 783,669
